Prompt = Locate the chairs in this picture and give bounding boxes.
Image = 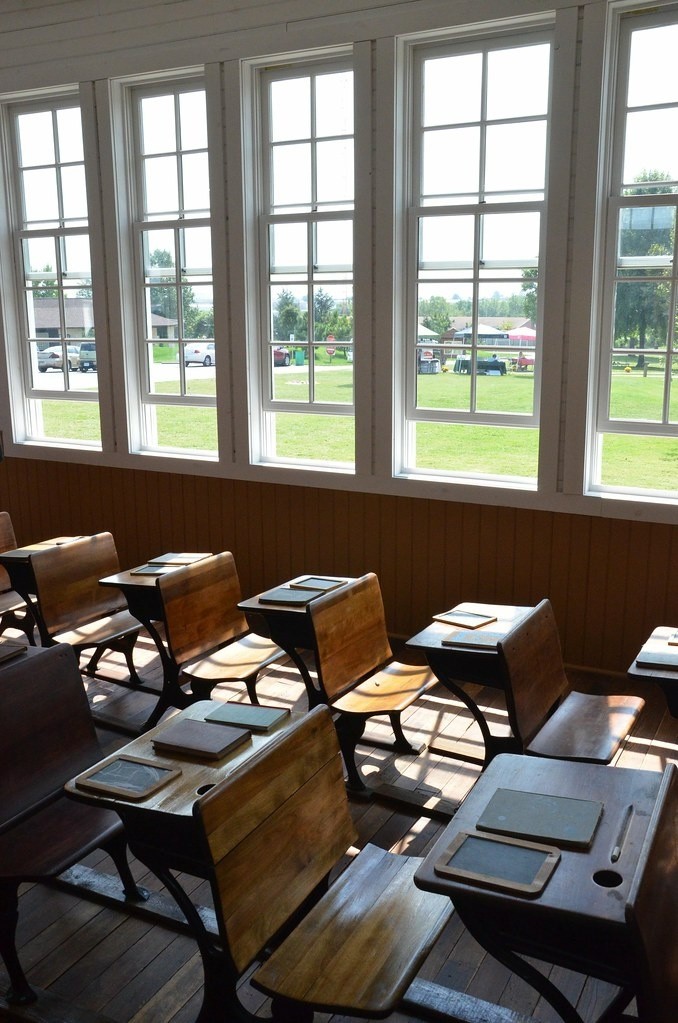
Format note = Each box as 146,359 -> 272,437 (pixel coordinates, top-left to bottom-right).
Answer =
306,571 -> 440,792
0,511 -> 38,647
0,642 -> 150,1007
624,762 -> 678,1023
497,599 -> 645,765
28,531 -> 158,685
191,704 -> 456,1023
155,551 -> 299,706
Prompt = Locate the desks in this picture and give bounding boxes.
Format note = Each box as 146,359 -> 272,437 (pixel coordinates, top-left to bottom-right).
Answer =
0,536 -> 91,647
98,552 -> 214,730
626,626 -> 678,720
236,575 -> 358,712
405,602 -> 559,813
414,752 -> 665,1023
64,700 -> 311,1023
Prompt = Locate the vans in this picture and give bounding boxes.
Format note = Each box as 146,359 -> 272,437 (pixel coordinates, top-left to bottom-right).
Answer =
78,342 -> 97,373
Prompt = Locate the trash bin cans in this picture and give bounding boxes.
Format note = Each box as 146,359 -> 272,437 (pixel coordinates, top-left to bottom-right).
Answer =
295,349 -> 305,366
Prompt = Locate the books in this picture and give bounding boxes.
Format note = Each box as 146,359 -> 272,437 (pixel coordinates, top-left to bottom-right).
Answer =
475,788 -> 605,851
204,701 -> 290,731
441,629 -> 506,650
147,553 -> 213,567
0,645 -> 27,663
636,652 -> 678,671
150,718 -> 252,760
258,588 -> 326,607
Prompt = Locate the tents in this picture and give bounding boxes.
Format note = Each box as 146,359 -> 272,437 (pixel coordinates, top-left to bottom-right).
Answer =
507,326 -> 536,373
455,325 -> 508,374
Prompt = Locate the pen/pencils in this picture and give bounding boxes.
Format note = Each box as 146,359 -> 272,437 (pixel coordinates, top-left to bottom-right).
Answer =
611,804 -> 633,862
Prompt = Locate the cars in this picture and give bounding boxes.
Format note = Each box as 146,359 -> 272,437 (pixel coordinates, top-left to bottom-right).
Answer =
37,345 -> 80,372
176,338 -> 216,367
274,345 -> 290,366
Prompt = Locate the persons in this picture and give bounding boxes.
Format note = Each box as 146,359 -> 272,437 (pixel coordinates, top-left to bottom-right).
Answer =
517,352 -> 527,371
488,354 -> 497,362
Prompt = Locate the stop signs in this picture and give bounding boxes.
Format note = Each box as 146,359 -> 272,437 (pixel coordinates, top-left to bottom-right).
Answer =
326,335 -> 336,355
